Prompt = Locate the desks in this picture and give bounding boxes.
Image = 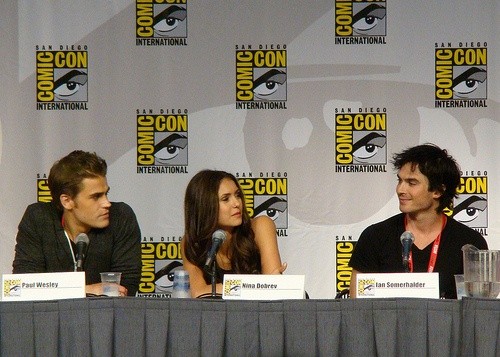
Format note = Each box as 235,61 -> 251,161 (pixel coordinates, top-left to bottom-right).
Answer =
0,296 -> 500,357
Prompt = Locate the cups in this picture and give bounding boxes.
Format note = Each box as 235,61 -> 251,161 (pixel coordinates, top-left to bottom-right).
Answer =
454,275 -> 466,299
100,272 -> 121,296
172,270 -> 192,298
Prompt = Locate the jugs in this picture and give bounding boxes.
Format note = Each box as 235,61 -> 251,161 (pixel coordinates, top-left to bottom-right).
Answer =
462,245 -> 500,298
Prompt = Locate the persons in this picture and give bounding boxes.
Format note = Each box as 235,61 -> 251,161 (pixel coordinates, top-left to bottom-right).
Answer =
180,169 -> 287,300
12,150 -> 142,298
348,145 -> 489,299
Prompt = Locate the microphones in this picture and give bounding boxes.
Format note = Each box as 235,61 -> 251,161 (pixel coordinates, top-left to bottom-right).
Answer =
400,231 -> 415,268
76,233 -> 89,268
204,230 -> 229,270
